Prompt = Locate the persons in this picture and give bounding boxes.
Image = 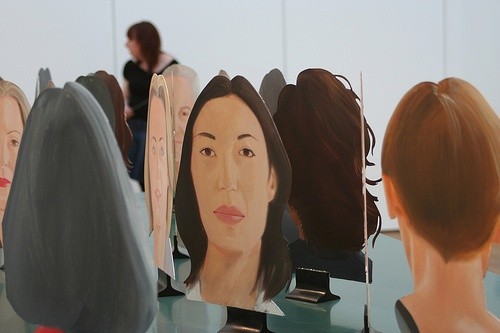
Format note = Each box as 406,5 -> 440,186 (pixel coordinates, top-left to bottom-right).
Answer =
162,64 -> 200,152
2,82 -> 157,333
142,73 -> 179,279
174,74 -> 294,316
120,22 -> 181,193
0,83 -> 31,248
380,77 -> 500,333
258,69 -> 287,120
273,68 -> 383,285
75,71 -> 135,176
